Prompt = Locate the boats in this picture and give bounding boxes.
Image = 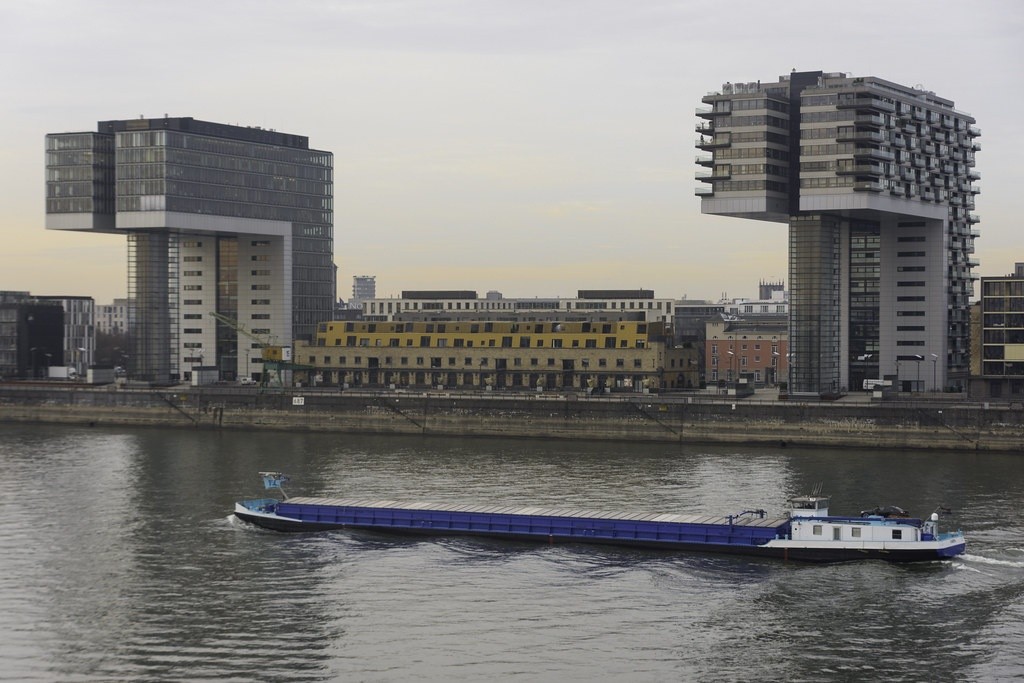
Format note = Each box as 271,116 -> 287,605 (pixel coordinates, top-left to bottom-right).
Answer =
232,472 -> 966,561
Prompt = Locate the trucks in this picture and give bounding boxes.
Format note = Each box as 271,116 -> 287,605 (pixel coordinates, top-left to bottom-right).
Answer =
48,366 -> 79,381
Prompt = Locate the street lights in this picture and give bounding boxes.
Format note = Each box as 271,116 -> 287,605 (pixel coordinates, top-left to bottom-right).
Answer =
728,352 -> 734,381
773,352 -> 780,388
198,349 -> 206,384
932,354 -> 938,395
864,354 -> 872,395
915,355 -> 922,391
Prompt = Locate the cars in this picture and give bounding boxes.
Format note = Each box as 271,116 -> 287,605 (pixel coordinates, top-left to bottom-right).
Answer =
241,378 -> 256,385
